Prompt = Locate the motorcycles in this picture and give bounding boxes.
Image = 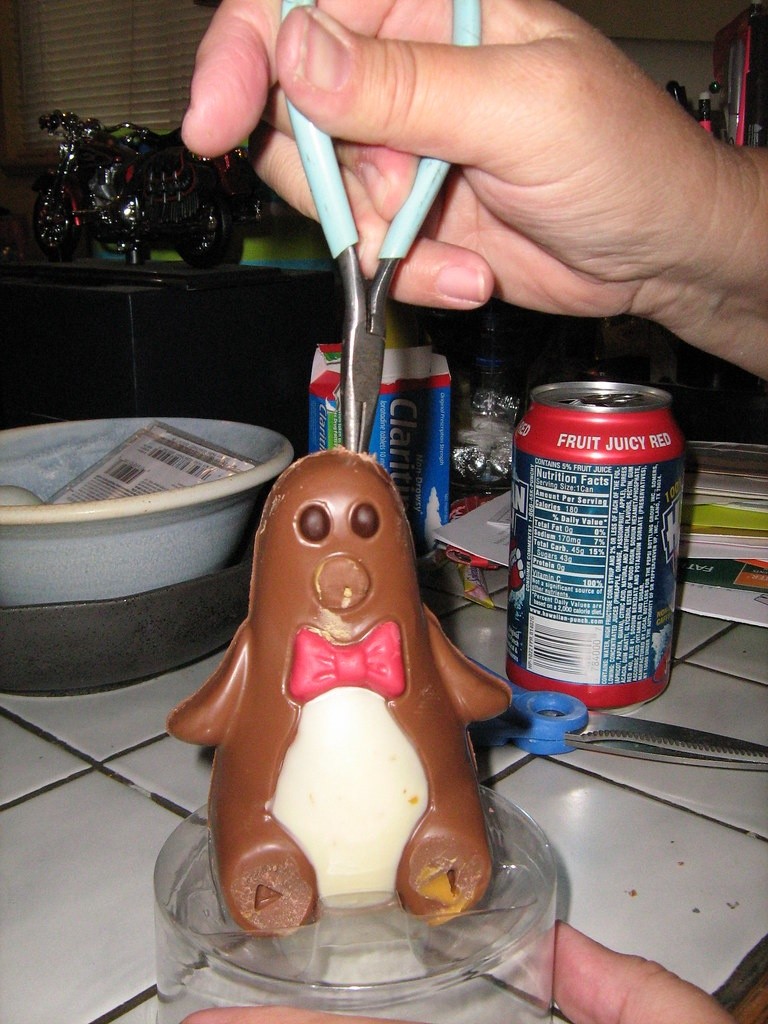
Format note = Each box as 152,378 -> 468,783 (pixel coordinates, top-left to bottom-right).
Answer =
26,109 -> 270,269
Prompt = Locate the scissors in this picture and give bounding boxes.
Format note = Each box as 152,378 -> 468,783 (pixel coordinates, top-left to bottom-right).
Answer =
465,656 -> 768,774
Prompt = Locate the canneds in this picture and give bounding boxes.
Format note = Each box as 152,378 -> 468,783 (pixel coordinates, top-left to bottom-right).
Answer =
507,380 -> 686,708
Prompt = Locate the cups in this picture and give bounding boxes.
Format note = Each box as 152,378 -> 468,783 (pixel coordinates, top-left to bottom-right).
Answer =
153,785 -> 559,1024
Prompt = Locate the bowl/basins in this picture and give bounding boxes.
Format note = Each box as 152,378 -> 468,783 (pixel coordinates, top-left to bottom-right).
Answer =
0,550 -> 253,691
0,417 -> 295,603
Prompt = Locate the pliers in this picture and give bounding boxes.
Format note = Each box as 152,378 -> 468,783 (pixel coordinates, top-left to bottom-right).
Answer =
281,0 -> 486,448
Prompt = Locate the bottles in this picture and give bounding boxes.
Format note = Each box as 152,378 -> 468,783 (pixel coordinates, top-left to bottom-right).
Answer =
697,99 -> 711,139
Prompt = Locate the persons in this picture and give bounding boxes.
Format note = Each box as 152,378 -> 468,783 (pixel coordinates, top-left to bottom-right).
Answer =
181,0 -> 768,1024
